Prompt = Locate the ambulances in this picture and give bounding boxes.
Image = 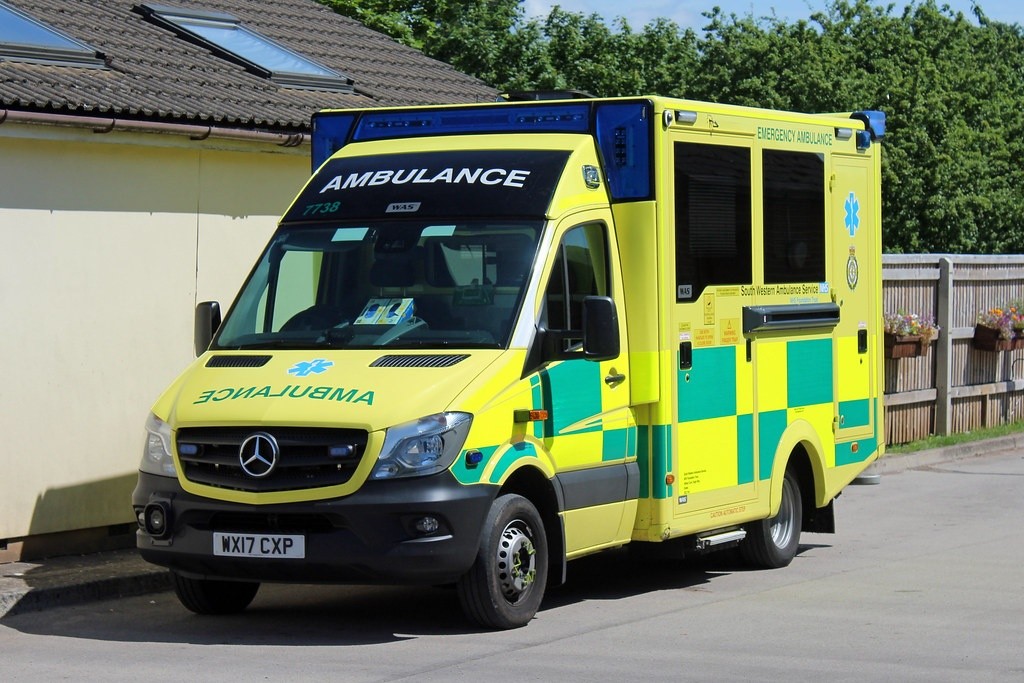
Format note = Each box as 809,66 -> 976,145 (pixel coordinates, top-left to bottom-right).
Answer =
129,91 -> 887,632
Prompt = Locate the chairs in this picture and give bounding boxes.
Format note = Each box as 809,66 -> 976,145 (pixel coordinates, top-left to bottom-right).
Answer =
468,244 -> 542,339
365,237 -> 448,323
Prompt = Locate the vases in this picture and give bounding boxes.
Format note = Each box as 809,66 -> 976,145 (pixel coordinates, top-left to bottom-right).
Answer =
975,323 -> 1024,352
883,331 -> 929,358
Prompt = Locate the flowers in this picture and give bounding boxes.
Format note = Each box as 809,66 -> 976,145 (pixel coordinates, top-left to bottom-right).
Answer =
883,307 -> 938,335
979,296 -> 1024,342
